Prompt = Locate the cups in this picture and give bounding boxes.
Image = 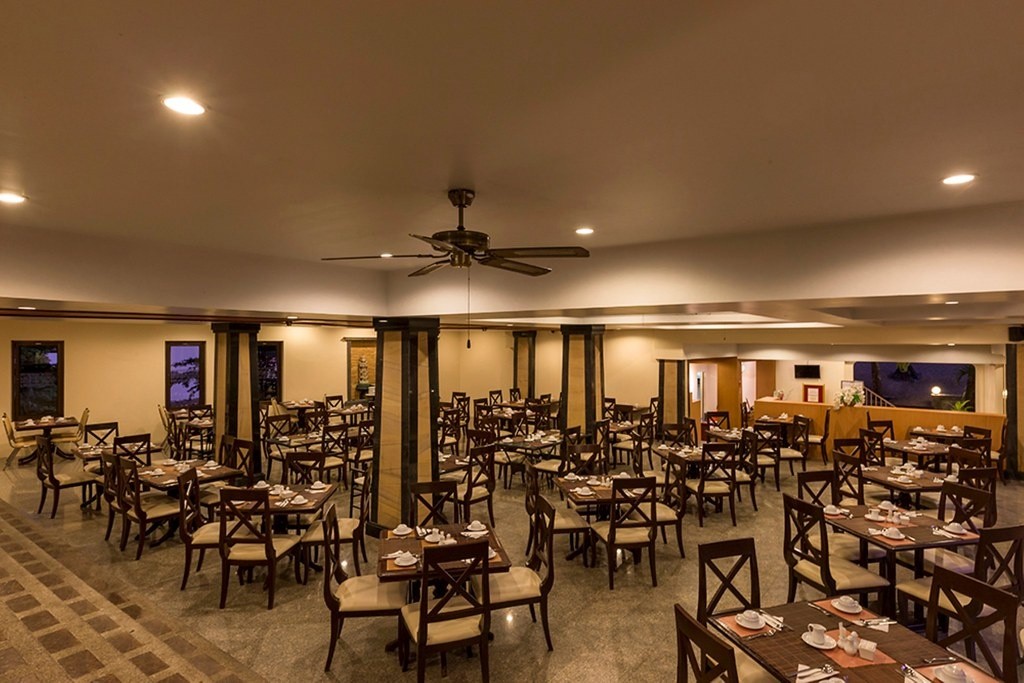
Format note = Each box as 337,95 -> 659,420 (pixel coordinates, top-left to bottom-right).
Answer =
294,495 -> 304,502
839,596 -> 857,610
736,610 -> 760,625
807,623 -> 827,645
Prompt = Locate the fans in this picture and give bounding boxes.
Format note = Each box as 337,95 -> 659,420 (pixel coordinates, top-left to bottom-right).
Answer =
321,187 -> 593,281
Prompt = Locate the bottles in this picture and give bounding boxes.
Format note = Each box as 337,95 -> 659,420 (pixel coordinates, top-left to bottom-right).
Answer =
837,621 -> 847,650
844,634 -> 858,656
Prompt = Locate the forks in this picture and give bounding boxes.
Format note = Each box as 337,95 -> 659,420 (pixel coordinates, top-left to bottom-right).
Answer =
895,668 -> 918,683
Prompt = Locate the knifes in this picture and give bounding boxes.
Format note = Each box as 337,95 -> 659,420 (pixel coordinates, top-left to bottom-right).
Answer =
784,661 -> 826,678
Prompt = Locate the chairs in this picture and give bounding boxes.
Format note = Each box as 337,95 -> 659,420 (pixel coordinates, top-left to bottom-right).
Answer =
0,388 -> 1024,683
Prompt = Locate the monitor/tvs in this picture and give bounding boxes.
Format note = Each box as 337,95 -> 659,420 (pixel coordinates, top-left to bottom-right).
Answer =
794,364 -> 820,378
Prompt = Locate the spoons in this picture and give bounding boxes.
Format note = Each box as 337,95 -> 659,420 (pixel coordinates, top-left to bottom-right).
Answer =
860,617 -> 890,623
864,620 -> 898,627
758,609 -> 796,631
923,657 -> 957,664
279,498 -> 292,508
900,663 -> 925,683
807,603 -> 832,616
797,664 -> 833,679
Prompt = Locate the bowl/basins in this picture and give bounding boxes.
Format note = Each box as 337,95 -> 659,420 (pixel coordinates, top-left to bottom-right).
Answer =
941,665 -> 967,682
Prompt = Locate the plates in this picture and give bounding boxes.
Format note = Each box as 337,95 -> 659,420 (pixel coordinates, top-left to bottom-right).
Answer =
379,517 -> 499,568
934,666 -> 975,683
735,613 -> 766,629
290,499 -> 308,504
801,631 -> 837,650
831,599 -> 862,613
822,499 -> 969,544
15,386 -> 970,495
